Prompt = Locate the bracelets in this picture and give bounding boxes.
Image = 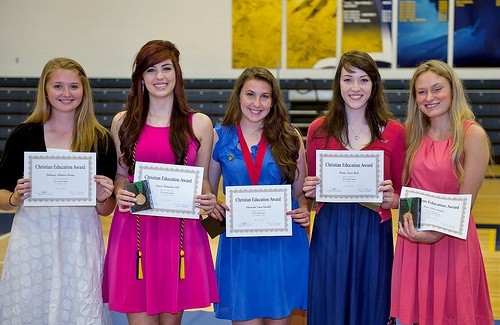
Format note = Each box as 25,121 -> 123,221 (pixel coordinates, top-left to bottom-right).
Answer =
96,196 -> 109,207
9,192 -> 18,206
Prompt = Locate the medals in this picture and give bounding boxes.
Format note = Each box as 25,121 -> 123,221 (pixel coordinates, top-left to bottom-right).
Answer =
135,192 -> 146,205
402,212 -> 414,222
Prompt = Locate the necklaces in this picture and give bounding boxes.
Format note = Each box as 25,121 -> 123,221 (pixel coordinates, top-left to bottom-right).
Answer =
347,121 -> 366,140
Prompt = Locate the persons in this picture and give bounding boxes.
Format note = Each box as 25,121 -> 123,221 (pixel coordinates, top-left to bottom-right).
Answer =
207,66 -> 311,325
0,57 -> 118,325
301,49 -> 407,325
390,59 -> 495,325
101,39 -> 221,324
